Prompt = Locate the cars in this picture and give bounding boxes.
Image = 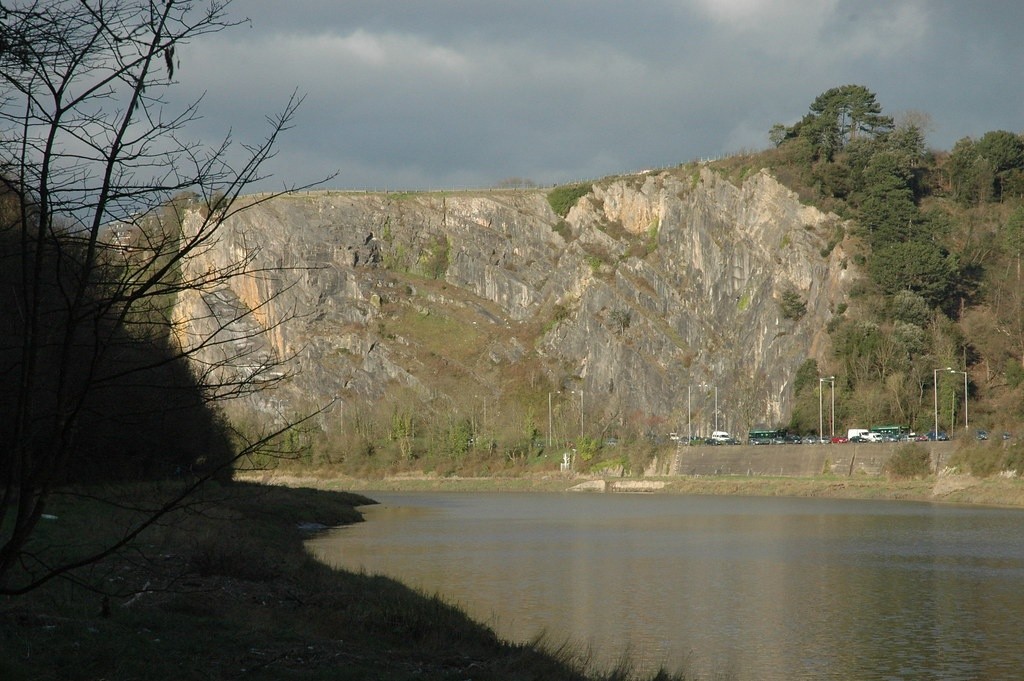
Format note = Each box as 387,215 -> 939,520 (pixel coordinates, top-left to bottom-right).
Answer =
667,430 -> 742,446
1002,431 -> 1010,440
977,428 -> 989,440
748,425 -> 949,445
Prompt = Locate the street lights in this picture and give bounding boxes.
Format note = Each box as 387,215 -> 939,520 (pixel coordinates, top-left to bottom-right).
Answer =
829,376 -> 835,436
549,391 -> 561,447
688,385 -> 702,446
475,395 -> 486,426
571,390 -> 583,438
820,377 -> 824,439
951,370 -> 969,431
934,367 -> 952,441
705,385 -> 717,442
